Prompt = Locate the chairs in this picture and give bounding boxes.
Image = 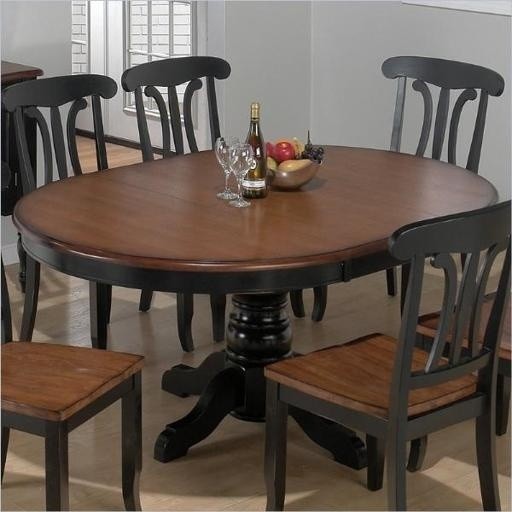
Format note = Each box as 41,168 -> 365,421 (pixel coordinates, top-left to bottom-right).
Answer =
0,74 -> 193,351
407,290 -> 512,472
262,200 -> 512,511
311,57 -> 504,323
1,256 -> 144,511
120,56 -> 307,351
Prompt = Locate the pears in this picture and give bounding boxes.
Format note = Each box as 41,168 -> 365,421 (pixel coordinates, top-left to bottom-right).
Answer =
278,159 -> 312,172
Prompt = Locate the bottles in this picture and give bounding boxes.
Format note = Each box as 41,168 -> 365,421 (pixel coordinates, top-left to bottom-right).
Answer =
240,102 -> 267,198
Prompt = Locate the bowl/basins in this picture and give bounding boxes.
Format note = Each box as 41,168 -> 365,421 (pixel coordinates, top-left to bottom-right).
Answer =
266,160 -> 324,189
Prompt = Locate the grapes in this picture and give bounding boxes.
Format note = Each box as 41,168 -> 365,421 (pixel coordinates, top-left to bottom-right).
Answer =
302,141 -> 324,164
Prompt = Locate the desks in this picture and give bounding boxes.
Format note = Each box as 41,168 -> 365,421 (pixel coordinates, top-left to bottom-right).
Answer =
1,61 -> 44,216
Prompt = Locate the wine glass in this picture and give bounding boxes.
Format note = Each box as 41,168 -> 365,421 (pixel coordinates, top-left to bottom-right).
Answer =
228,142 -> 255,208
215,136 -> 240,201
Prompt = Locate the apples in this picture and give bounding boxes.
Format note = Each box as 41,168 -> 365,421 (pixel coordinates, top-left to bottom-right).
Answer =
267,156 -> 277,172
273,143 -> 295,161
265,143 -> 274,159
